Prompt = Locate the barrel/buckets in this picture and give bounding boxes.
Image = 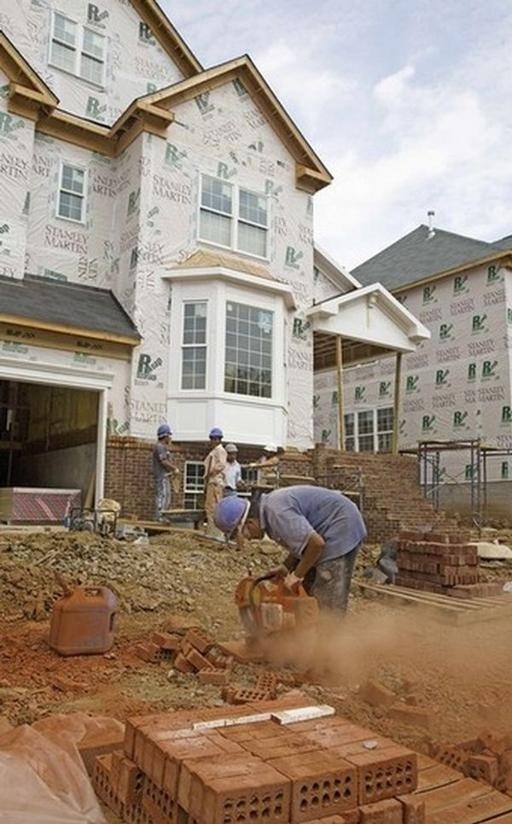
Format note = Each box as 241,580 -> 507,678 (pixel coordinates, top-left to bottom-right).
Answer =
50,585 -> 118,656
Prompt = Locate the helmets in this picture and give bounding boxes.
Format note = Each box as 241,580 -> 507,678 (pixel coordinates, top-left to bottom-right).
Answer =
213,495 -> 248,538
209,428 -> 224,437
158,425 -> 172,434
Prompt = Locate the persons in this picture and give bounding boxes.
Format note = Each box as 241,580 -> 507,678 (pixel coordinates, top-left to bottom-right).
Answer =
153,424 -> 179,523
213,485 -> 367,616
203,428 -> 244,537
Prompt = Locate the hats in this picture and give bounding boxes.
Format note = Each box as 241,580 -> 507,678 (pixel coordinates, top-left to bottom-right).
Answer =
225,443 -> 239,453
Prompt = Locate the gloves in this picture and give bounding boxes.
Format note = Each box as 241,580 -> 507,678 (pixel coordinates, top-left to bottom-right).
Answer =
269,564 -> 305,590
